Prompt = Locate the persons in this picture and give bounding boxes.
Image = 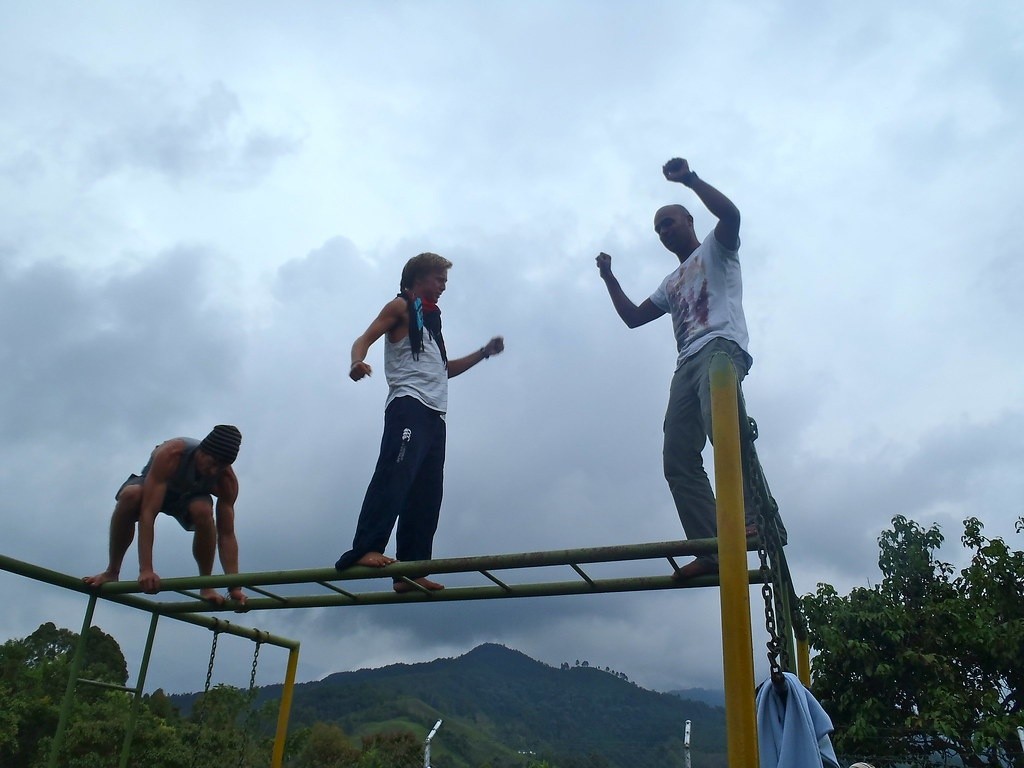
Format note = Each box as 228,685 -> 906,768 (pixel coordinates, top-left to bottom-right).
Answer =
339,253 -> 504,592
596,157 -> 788,578
80,424 -> 252,614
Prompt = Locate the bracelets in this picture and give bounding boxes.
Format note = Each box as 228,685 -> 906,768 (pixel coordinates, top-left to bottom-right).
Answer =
350,360 -> 362,368
683,171 -> 697,188
227,586 -> 241,592
481,348 -> 490,359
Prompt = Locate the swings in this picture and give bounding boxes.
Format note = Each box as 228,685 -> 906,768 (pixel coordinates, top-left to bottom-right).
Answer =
180,630 -> 270,768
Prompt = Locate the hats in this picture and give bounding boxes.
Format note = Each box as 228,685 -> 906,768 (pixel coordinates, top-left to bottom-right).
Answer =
199,425 -> 242,464
